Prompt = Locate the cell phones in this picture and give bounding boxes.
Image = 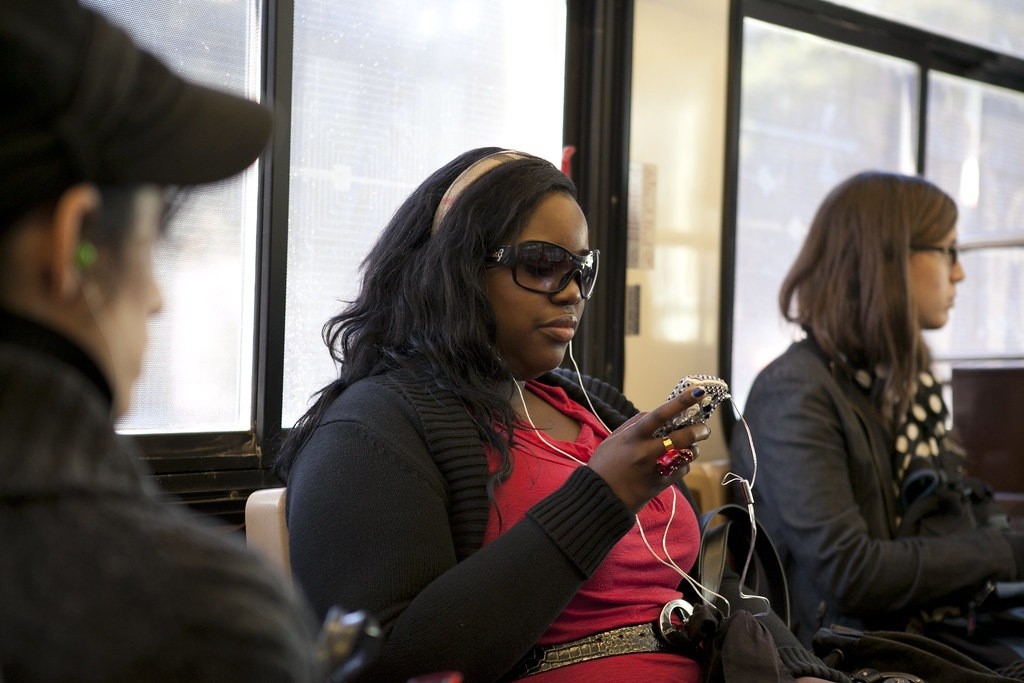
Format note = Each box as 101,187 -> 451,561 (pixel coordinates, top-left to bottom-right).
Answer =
653,374 -> 731,440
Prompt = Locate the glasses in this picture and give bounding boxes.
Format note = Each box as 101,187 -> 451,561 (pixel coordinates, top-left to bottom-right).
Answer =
487,239 -> 600,299
913,244 -> 959,264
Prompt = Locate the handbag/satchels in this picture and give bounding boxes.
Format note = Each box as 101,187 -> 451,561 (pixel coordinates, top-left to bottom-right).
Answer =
684,504 -> 797,683
897,467 -> 1008,631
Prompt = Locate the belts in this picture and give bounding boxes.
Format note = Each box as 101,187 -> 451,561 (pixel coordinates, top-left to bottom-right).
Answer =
494,597 -> 695,683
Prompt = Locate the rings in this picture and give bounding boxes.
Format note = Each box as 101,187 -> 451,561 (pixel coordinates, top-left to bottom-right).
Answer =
662,436 -> 675,451
657,449 -> 693,477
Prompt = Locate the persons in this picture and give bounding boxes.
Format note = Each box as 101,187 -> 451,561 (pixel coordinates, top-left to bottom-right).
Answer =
282,146 -> 856,683
729,169 -> 1024,683
0,1 -> 337,683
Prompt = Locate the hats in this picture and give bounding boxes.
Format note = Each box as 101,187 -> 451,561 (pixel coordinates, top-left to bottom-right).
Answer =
0,0 -> 272,224
